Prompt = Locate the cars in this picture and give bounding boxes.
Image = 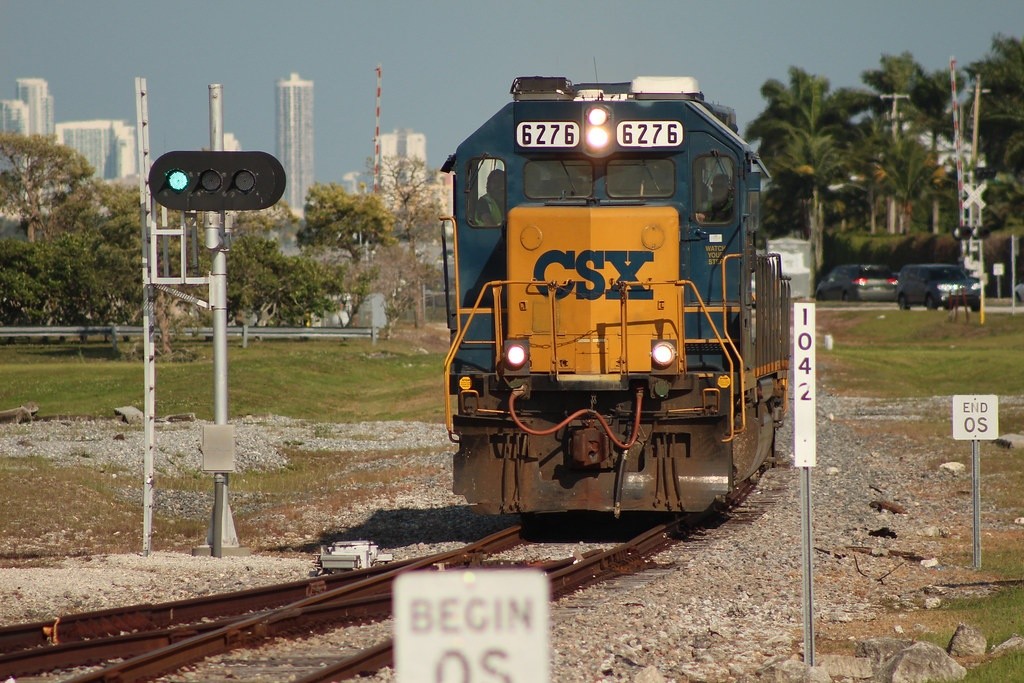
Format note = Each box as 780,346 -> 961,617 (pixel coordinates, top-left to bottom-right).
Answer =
815,264 -> 899,304
1011,283 -> 1024,304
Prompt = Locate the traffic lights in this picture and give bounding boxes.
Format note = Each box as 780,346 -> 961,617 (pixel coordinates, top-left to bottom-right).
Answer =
148,149 -> 285,210
974,226 -> 990,240
954,227 -> 972,239
977,167 -> 996,181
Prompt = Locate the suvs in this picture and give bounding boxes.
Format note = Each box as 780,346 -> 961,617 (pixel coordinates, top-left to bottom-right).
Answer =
894,263 -> 981,313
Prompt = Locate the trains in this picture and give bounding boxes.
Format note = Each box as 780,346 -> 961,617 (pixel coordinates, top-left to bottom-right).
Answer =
440,74 -> 794,533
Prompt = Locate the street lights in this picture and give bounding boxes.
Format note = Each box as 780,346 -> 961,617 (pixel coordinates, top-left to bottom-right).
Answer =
827,182 -> 874,236
